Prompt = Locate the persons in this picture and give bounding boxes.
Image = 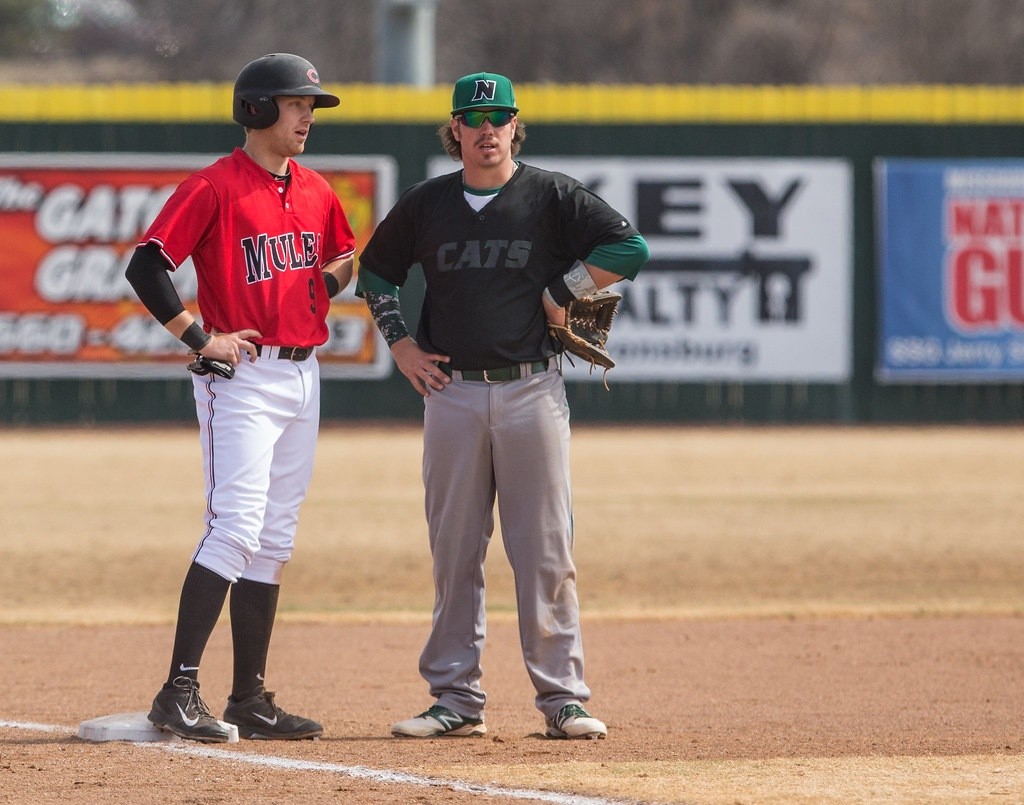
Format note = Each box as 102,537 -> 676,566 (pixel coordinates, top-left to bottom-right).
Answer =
123,53 -> 354,744
356,73 -> 651,739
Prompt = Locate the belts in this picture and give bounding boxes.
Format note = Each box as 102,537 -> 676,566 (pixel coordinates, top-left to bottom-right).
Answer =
437,363 -> 547,385
246,344 -> 314,362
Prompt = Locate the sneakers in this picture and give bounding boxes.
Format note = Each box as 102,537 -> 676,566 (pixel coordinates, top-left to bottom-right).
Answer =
392,705 -> 486,737
147,676 -> 230,743
224,686 -> 323,740
545,704 -> 607,739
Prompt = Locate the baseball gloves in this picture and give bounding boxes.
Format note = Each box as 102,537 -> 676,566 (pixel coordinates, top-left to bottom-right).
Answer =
549,289 -> 622,372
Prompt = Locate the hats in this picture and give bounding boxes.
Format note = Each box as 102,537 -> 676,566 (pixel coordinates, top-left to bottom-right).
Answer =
451,72 -> 519,113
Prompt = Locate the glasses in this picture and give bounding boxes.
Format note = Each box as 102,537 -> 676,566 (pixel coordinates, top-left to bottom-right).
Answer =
460,110 -> 513,127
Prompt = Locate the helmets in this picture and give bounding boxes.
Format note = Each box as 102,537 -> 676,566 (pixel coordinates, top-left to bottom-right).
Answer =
233,53 -> 340,128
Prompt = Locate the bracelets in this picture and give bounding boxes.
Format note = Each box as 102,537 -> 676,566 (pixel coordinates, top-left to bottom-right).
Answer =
548,273 -> 576,308
322,272 -> 339,299
179,320 -> 212,353
